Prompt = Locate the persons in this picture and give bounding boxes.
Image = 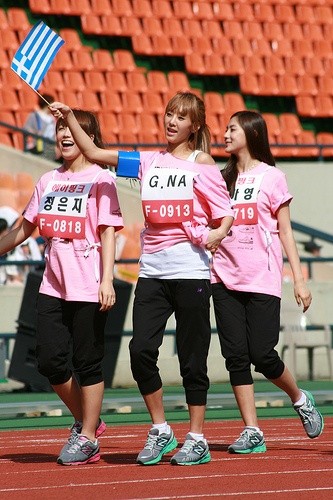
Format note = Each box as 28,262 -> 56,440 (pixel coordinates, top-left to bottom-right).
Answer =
297,239 -> 320,281
0,108 -> 125,466
0,208 -> 46,285
208,110 -> 324,454
47,91 -> 234,469
23,92 -> 58,156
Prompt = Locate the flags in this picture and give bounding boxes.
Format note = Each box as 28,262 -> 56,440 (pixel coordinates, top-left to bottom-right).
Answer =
9,19 -> 64,90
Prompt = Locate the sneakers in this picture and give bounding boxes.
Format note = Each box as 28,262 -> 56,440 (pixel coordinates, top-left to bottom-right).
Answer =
56,433 -> 101,465
137,425 -> 178,465
292,388 -> 324,440
58,417 -> 107,455
170,432 -> 211,465
227,427 -> 266,454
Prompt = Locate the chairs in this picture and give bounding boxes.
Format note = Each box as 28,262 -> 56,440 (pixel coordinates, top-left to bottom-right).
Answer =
1,0 -> 333,163
